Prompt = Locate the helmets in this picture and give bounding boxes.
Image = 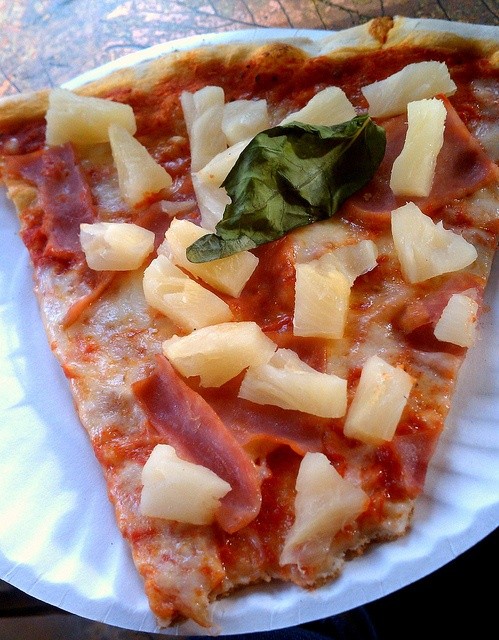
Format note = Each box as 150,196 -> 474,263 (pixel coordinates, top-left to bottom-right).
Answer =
1,17 -> 499,634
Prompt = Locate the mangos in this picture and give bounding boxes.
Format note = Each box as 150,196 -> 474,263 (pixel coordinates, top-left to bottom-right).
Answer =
0,15 -> 499,626
